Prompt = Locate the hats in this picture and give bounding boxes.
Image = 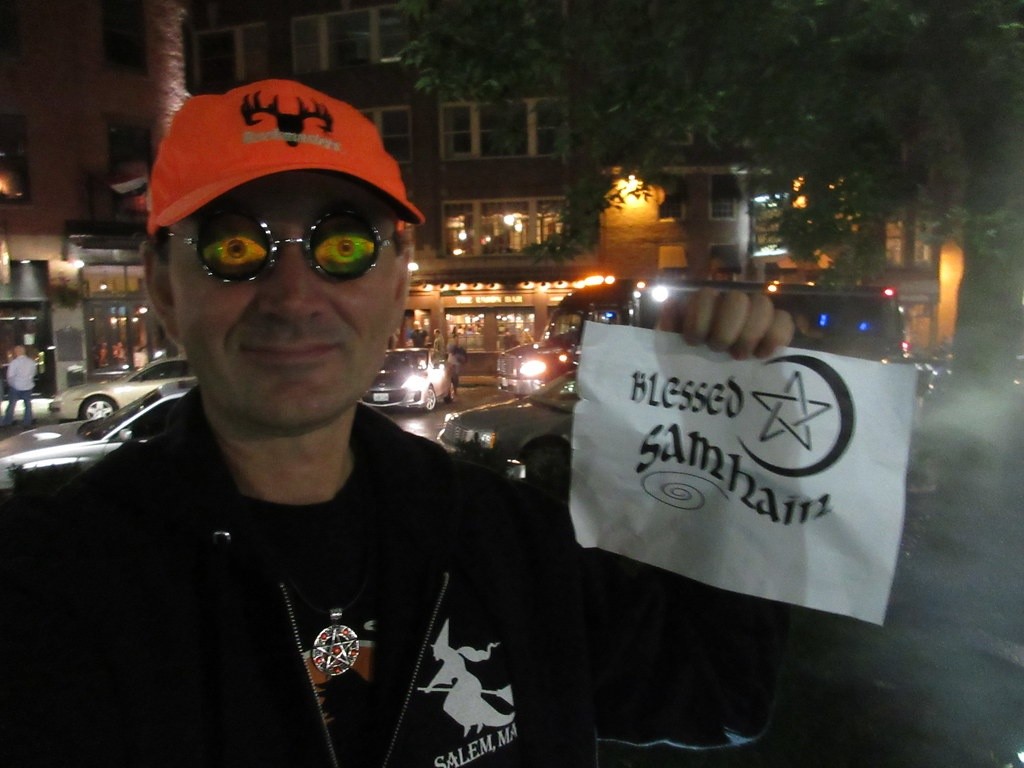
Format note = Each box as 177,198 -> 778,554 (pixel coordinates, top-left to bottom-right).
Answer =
146,78 -> 426,238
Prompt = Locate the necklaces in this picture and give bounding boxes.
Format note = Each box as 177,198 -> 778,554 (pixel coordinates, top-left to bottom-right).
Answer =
290,570 -> 372,675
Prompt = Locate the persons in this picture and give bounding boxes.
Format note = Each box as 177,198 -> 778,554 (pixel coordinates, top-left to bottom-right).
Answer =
411,321 -> 467,396
4,345 -> 37,427
0,79 -> 794,767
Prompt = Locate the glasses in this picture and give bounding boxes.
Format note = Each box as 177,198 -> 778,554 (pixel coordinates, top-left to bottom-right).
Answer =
161,206 -> 395,281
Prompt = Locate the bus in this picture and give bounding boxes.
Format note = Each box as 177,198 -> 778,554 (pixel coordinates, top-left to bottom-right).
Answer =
496,274 -> 910,396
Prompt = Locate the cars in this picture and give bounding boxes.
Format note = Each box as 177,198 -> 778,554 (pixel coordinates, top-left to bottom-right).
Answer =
0,380 -> 196,492
438,371 -> 612,502
357,347 -> 455,413
46,358 -> 198,422
